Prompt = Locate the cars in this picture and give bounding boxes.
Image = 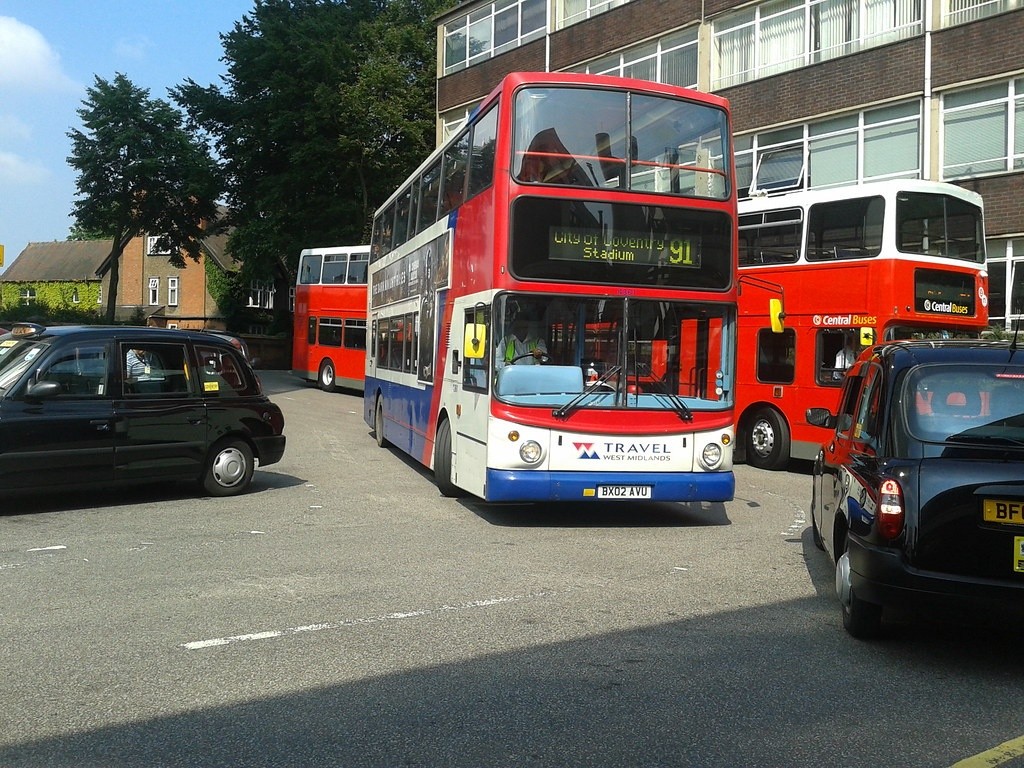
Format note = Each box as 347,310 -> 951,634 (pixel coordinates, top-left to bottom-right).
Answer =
0,321 -> 286,497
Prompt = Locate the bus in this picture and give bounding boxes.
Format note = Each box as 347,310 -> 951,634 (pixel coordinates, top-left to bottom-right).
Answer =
291,244 -> 417,394
555,178 -> 990,471
364,71 -> 787,505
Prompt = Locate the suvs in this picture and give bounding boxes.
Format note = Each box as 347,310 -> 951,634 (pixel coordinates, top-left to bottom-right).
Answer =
804,339 -> 1023,637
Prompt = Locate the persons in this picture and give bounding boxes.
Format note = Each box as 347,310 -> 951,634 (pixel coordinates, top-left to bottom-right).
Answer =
495,313 -> 549,373
831,336 -> 860,380
126,348 -> 159,379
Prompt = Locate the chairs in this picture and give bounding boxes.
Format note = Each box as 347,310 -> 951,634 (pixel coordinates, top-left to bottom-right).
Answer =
918,383 -> 982,438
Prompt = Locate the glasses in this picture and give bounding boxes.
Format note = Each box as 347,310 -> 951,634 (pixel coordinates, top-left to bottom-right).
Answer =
512,326 -> 530,332
